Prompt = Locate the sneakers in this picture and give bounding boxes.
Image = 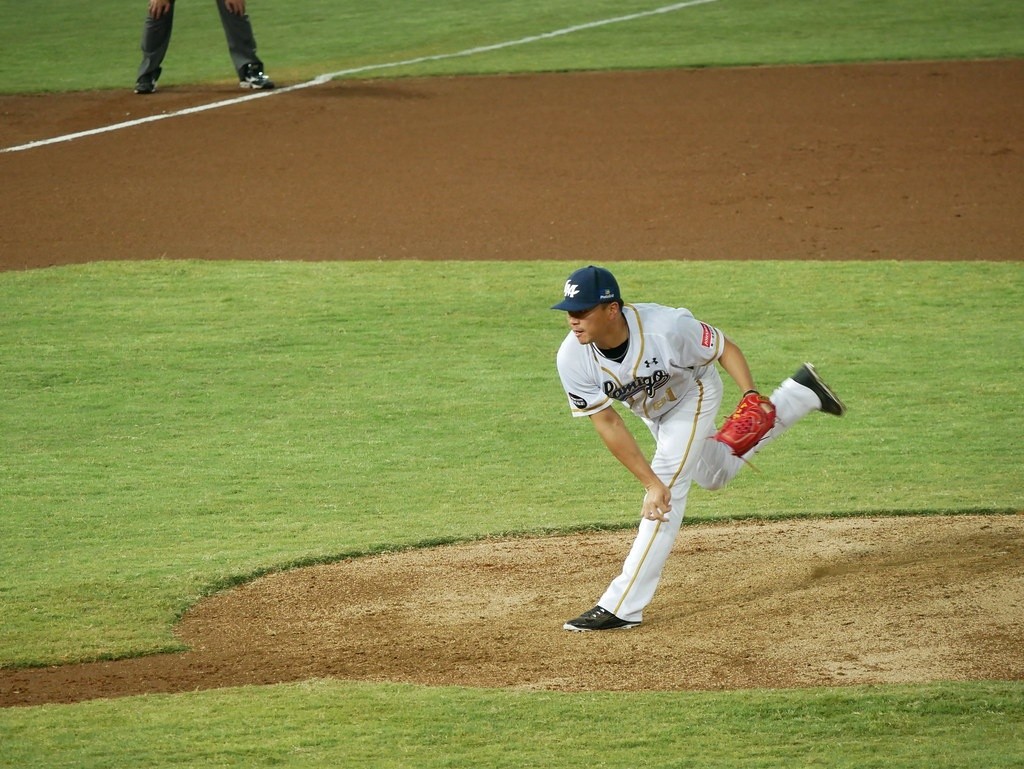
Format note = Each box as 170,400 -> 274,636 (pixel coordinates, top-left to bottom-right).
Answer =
239,63 -> 275,90
134,77 -> 158,94
790,361 -> 847,416
562,605 -> 641,633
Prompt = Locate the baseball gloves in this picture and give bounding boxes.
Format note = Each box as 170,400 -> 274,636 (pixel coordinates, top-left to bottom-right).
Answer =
713,394 -> 777,457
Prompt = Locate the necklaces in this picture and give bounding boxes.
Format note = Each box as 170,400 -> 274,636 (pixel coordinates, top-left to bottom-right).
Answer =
592,332 -> 630,359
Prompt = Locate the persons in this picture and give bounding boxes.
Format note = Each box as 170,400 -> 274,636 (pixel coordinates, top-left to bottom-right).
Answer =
550,265 -> 845,632
133,1 -> 275,93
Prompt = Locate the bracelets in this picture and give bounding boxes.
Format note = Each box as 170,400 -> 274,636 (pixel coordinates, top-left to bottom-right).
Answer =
743,390 -> 760,398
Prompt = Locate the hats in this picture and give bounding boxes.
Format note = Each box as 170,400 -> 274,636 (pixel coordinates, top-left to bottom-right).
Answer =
550,265 -> 620,311
